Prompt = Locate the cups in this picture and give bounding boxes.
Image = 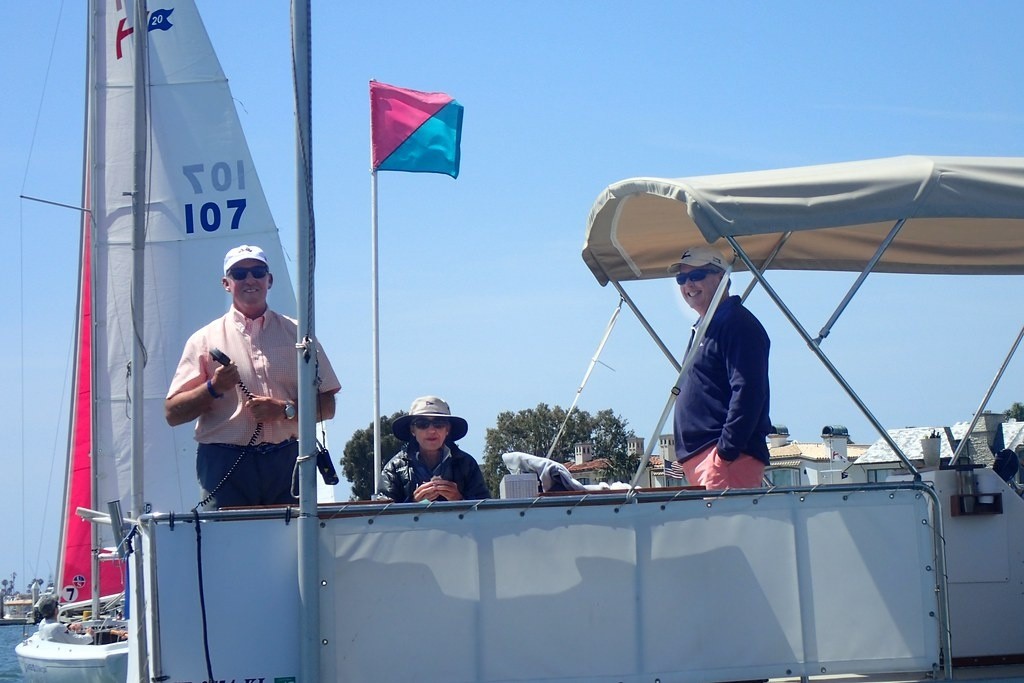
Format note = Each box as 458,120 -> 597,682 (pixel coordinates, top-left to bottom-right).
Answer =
921,438 -> 941,467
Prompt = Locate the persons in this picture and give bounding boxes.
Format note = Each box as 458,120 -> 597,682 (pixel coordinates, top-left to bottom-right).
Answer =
377,395 -> 491,502
166,245 -> 342,510
994,449 -> 1024,499
109,614 -> 128,643
667,244 -> 771,490
38,598 -> 94,645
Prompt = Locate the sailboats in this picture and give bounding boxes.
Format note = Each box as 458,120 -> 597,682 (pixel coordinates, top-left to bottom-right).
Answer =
15,1 -> 333,683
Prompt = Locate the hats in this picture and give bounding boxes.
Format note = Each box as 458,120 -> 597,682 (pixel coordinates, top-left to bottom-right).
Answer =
667,247 -> 730,273
223,245 -> 270,277
392,397 -> 469,442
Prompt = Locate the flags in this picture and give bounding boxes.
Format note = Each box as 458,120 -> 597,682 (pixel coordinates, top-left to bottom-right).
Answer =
369,81 -> 465,180
665,459 -> 683,479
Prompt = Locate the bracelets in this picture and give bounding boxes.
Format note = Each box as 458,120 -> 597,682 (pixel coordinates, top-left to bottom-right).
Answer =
207,379 -> 224,399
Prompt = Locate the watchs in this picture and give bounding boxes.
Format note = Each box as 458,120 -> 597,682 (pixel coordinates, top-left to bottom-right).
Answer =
284,400 -> 295,420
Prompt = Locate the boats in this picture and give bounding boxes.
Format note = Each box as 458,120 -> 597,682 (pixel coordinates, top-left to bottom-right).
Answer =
75,157 -> 1024,669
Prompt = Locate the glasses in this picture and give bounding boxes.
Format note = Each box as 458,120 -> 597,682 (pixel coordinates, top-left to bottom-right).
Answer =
412,418 -> 449,429
227,266 -> 268,281
675,268 -> 720,285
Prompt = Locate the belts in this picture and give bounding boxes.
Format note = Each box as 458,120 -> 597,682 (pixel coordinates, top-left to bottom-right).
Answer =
211,438 -> 297,455
